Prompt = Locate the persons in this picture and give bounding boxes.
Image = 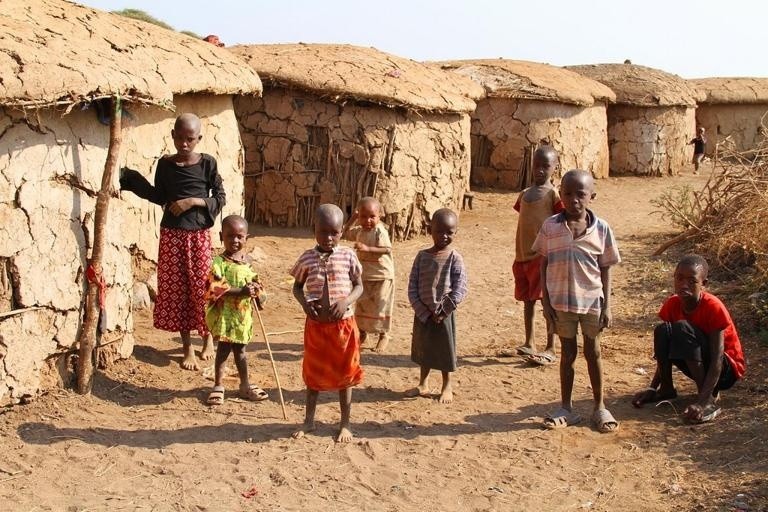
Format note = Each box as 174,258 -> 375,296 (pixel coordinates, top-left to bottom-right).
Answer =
403,208 -> 467,403
353,197 -> 393,352
288,204 -> 364,442
501,145 -> 565,366
531,169 -> 621,432
119,112 -> 226,370
632,254 -> 747,423
203,216 -> 267,404
686,127 -> 707,175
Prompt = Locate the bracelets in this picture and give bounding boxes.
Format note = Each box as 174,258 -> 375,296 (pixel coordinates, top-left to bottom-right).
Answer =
647,388 -> 656,391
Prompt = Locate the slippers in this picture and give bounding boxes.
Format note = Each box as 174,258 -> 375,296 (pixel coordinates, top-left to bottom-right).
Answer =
689,406 -> 720,423
531,353 -> 556,365
542,409 -> 581,429
641,400 -> 676,407
502,346 -> 534,357
591,409 -> 618,433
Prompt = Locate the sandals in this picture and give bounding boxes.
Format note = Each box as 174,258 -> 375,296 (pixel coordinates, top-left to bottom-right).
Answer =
206,387 -> 225,404
240,383 -> 268,402
